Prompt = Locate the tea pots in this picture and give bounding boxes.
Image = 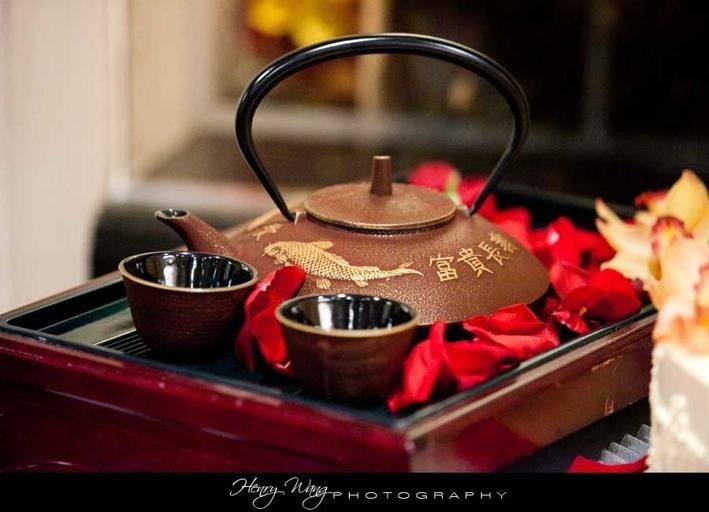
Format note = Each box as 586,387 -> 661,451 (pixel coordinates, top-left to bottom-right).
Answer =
154,34 -> 548,330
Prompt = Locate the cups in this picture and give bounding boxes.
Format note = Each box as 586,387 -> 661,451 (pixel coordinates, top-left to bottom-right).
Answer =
121,250 -> 257,353
275,293 -> 417,391
